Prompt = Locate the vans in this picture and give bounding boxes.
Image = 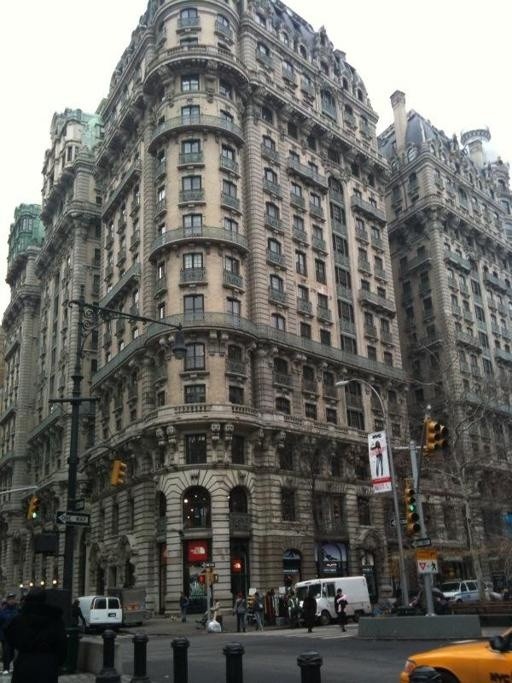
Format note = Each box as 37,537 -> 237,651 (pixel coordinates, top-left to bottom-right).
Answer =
288,575 -> 373,625
77,595 -> 123,632
440,579 -> 482,608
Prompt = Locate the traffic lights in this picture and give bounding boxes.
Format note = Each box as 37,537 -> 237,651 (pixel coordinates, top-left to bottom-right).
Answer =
197,573 -> 219,584
403,487 -> 421,535
110,460 -> 128,487
426,419 -> 450,452
25,495 -> 41,521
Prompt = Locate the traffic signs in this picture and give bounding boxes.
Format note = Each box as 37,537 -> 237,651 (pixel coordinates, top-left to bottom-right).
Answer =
56,511 -> 90,528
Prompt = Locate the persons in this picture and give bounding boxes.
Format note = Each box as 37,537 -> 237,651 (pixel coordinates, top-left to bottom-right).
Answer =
211,598 -> 223,632
337,594 -> 347,613
180,592 -> 189,623
371,442 -> 385,476
334,588 -> 348,632
303,590 -> 317,633
233,590 -> 302,632
0,586 -> 86,683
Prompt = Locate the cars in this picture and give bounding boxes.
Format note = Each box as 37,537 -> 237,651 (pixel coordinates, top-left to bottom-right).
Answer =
399,624 -> 512,683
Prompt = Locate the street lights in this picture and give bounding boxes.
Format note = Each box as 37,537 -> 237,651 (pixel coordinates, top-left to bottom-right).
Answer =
333,377 -> 417,617
